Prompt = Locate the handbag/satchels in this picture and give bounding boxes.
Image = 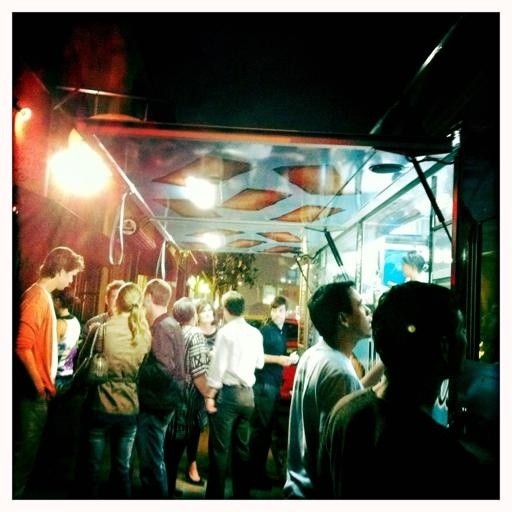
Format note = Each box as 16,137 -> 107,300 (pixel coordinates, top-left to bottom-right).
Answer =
84,322 -> 110,384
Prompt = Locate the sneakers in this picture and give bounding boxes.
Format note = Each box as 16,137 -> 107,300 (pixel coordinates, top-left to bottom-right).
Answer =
184,469 -> 205,487
